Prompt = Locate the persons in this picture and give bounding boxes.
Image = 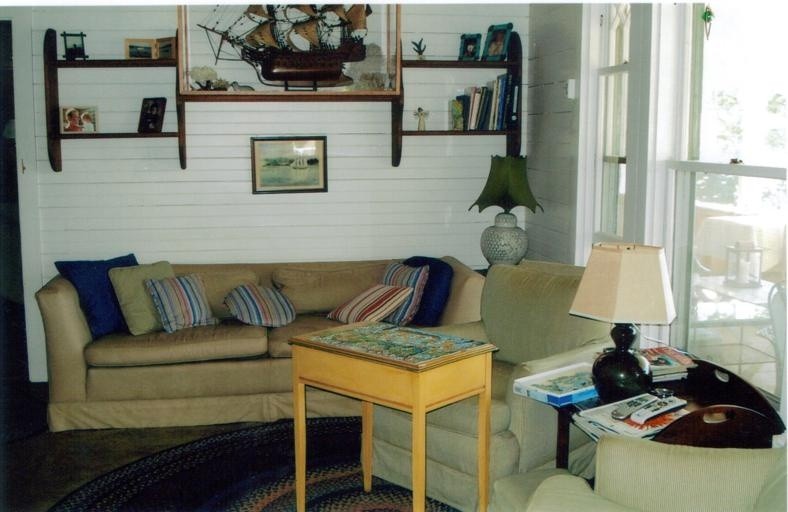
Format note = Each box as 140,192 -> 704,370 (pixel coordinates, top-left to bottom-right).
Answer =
142,103 -> 161,131
80,112 -> 95,132
63,108 -> 85,132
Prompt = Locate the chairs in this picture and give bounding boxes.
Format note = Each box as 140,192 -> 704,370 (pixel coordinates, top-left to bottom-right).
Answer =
360,259 -> 614,512
486,400 -> 785,512
765,281 -> 786,393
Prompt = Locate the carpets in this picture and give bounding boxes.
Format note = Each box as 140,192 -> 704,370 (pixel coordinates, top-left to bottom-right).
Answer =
45,418 -> 462,512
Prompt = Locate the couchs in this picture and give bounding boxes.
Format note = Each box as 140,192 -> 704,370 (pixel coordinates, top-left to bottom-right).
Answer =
33,255 -> 484,436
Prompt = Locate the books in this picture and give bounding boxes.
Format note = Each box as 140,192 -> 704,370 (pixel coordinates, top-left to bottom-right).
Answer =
571,346 -> 698,444
449,73 -> 509,131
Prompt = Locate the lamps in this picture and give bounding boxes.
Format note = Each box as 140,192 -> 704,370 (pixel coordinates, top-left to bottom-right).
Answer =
565,239 -> 678,403
466,154 -> 544,269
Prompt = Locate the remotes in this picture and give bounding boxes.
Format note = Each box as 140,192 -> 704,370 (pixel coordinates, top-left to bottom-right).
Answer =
611,395 -> 658,420
630,396 -> 688,425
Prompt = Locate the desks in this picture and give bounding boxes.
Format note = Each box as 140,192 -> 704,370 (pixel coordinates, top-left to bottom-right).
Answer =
286,321 -> 500,512
694,210 -> 786,271
548,358 -> 784,470
696,270 -> 777,391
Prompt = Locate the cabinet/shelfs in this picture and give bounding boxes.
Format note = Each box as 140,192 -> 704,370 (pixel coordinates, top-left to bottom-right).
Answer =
391,30 -> 522,169
42,27 -> 186,169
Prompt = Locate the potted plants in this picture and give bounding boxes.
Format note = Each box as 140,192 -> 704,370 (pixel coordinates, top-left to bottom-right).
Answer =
412,37 -> 427,61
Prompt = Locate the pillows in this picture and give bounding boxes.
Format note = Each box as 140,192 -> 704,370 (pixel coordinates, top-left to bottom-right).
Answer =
51,252 -> 140,341
222,282 -> 295,329
141,269 -> 221,334
107,259 -> 176,337
325,282 -> 414,325
377,260 -> 430,327
402,255 -> 454,328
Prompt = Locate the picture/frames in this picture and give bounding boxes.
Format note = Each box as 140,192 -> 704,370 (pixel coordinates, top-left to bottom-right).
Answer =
57,103 -> 101,134
457,32 -> 481,60
481,22 -> 513,64
59,30 -> 90,62
251,135 -> 328,195
177,4 -> 403,103
138,95 -> 167,131
125,34 -> 177,60
